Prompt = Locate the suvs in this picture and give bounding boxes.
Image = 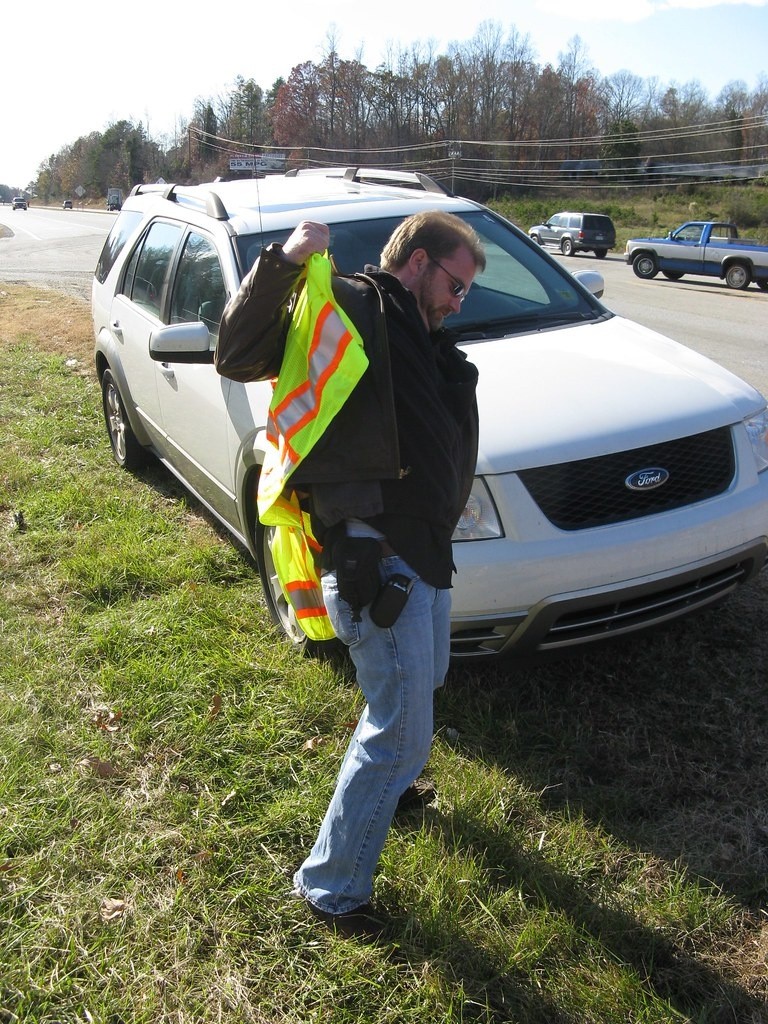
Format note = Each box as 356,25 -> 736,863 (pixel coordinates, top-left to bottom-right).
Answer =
526,211 -> 615,259
12,197 -> 27,210
62,200 -> 73,208
89,165 -> 768,658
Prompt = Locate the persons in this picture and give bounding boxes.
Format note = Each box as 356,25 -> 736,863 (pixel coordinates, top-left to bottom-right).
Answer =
214,212 -> 486,943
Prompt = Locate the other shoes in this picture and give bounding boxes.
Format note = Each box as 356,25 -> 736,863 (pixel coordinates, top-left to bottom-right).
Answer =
309,900 -> 384,942
400,782 -> 437,809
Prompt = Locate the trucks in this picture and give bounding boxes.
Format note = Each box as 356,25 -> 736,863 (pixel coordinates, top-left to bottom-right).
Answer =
106,188 -> 123,210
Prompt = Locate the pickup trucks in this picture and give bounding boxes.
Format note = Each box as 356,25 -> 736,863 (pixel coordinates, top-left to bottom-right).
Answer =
625,221 -> 768,290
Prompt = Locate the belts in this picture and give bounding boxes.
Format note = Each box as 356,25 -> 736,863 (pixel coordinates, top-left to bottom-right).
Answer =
379,541 -> 397,557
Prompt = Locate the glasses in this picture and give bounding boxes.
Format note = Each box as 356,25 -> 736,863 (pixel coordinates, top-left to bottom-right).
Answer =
427,253 -> 467,304
